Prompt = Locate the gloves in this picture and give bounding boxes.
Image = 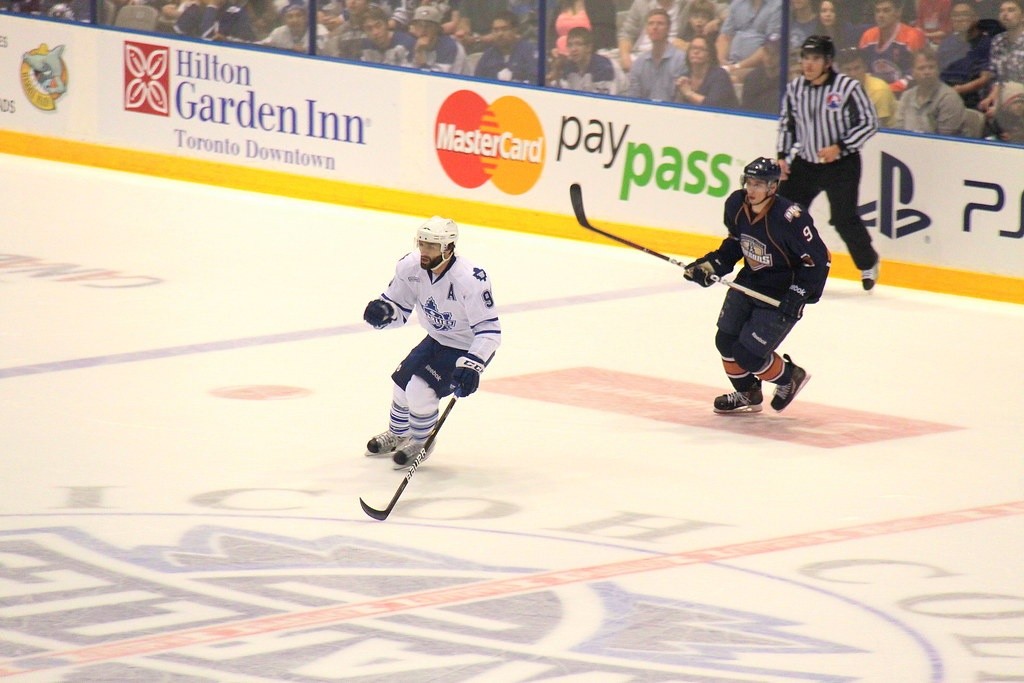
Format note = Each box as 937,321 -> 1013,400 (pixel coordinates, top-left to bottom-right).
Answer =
364,300 -> 397,327
768,279 -> 814,320
682,250 -> 734,287
449,353 -> 486,398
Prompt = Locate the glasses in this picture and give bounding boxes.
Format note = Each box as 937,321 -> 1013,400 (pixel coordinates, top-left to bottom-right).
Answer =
688,45 -> 711,52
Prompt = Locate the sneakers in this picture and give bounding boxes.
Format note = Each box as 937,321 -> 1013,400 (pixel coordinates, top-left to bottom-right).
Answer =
770,352 -> 811,413
393,437 -> 435,470
364,429 -> 411,457
713,377 -> 763,412
862,254 -> 879,290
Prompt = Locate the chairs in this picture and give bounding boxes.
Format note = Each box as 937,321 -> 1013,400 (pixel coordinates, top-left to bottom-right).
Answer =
454,30 -> 988,139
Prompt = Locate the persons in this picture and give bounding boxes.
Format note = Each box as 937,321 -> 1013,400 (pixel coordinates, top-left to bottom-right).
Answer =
682,155 -> 831,411
775,36 -> 880,293
363,215 -> 502,465
1,2 -> 1024,146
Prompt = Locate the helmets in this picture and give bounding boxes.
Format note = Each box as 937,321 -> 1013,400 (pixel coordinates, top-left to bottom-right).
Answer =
744,157 -> 780,185
413,216 -> 459,253
801,34 -> 835,58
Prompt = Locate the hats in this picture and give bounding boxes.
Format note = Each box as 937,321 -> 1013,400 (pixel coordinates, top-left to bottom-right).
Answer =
1001,80 -> 1024,112
412,5 -> 443,27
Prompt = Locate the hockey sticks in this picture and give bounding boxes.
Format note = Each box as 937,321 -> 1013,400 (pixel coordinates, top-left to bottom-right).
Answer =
358,350 -> 498,522
567,181 -> 785,307
